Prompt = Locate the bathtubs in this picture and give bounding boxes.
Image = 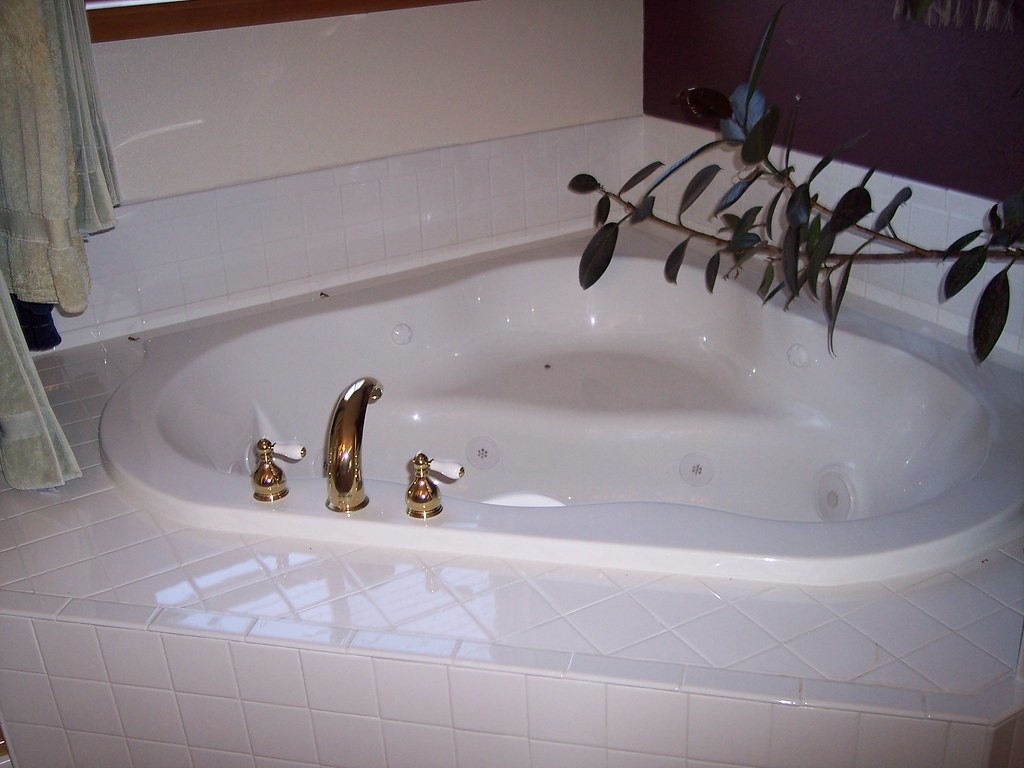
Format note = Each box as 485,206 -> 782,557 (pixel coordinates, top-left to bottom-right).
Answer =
96,253 -> 1024,583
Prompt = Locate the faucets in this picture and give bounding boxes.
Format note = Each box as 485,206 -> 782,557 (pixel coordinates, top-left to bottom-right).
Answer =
324,376 -> 384,513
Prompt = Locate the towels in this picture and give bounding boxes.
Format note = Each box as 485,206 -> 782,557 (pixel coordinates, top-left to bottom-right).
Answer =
0,269 -> 84,494
0,0 -> 121,354
